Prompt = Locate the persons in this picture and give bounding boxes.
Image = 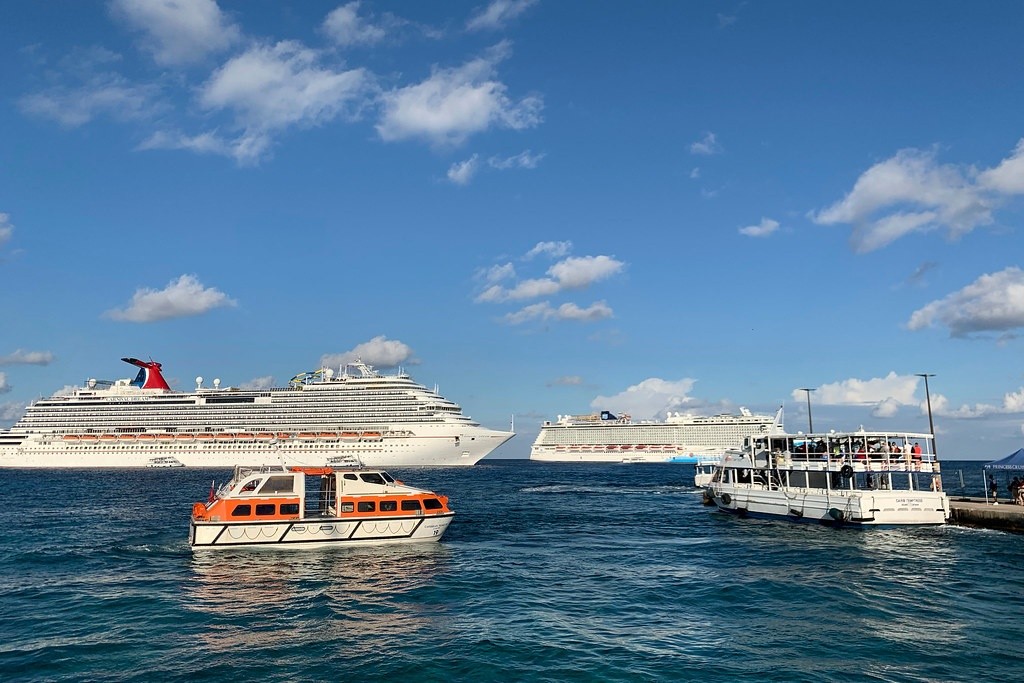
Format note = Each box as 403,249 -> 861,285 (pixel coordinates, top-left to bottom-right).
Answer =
989,475 -> 1024,506
798,439 -> 922,464
866,473 -> 885,489
930,478 -> 941,492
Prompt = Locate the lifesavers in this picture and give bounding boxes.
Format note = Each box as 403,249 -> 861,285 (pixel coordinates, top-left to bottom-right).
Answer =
720,494 -> 731,505
829,509 -> 844,521
841,465 -> 853,479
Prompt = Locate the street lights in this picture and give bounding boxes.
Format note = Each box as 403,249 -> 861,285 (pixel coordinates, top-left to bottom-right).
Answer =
800,388 -> 817,435
915,373 -> 939,463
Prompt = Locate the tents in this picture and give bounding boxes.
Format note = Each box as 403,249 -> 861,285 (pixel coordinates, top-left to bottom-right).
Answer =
982,448 -> 1024,506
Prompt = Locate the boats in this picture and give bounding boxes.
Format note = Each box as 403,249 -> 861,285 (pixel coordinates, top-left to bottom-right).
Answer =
80,436 -> 98,444
635,445 -> 648,452
338,431 -> 361,443
214,434 -> 235,443
296,432 -> 317,442
606,445 -> 619,452
155,434 -> 174,443
234,433 -> 256,442
553,445 -> 567,452
315,432 -> 339,443
703,404 -> 955,530
276,431 -> 294,442
175,434 -> 194,443
63,436 -> 79,444
195,434 -> 214,443
650,446 -> 662,451
694,460 -> 723,489
360,431 -> 385,443
136,435 -> 155,443
664,446 -> 676,451
117,435 -> 136,443
324,455 -> 363,470
594,445 -> 605,452
620,445 -> 633,451
581,446 -> 592,451
147,457 -> 184,468
99,435 -> 116,443
187,466 -> 455,553
568,445 -> 580,451
254,434 -> 274,443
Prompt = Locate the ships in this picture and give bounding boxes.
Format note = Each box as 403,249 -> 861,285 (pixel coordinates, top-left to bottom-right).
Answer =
528,405 -> 786,467
2,357 -> 519,472
